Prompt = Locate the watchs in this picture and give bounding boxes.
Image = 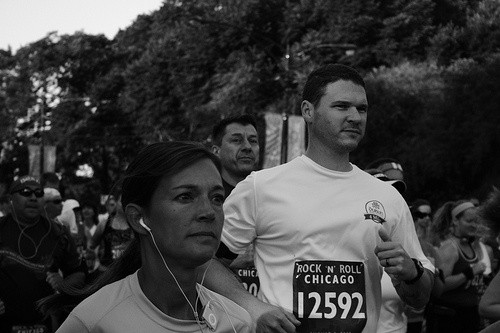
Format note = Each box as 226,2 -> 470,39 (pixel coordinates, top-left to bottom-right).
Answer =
404,258 -> 424,285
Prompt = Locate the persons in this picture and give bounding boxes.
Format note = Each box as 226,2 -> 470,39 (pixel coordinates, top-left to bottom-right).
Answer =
55,142 -> 256,333
197,64 -> 435,333
0,172 -> 136,333
209,113 -> 261,296
362,159 -> 500,333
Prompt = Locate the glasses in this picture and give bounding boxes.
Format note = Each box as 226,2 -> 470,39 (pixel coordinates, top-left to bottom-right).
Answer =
415,211 -> 431,219
10,187 -> 44,198
44,199 -> 62,205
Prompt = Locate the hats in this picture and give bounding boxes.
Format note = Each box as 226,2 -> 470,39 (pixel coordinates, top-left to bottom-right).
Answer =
11,175 -> 42,192
365,168 -> 407,195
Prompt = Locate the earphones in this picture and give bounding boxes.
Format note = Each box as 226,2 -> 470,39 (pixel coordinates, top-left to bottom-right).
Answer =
137,216 -> 152,231
9,199 -> 13,204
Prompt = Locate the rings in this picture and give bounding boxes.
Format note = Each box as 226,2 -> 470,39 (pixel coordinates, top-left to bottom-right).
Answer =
386,258 -> 390,267
